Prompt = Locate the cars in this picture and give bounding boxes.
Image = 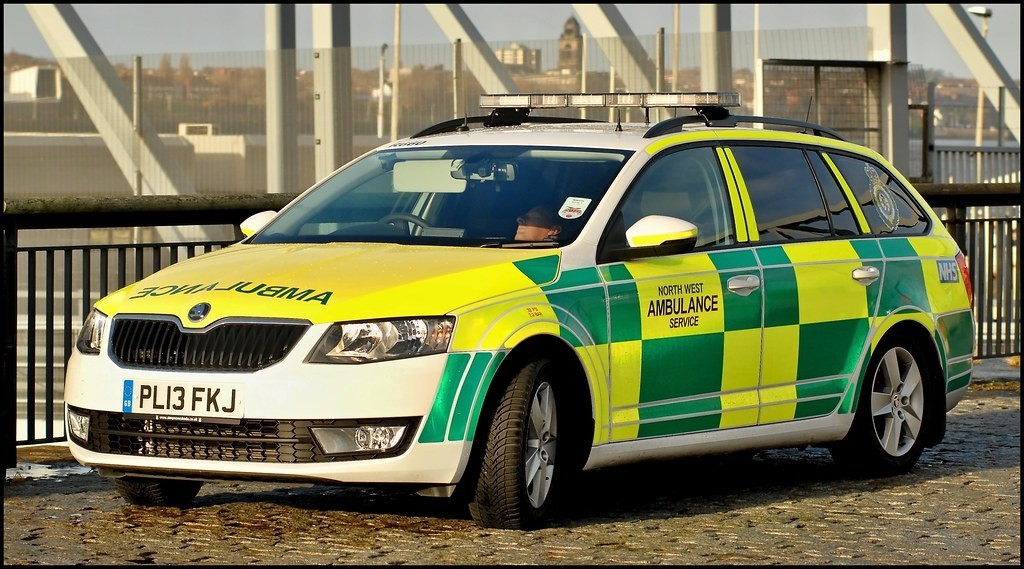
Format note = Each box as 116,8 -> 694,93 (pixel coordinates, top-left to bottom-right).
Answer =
60,91 -> 978,531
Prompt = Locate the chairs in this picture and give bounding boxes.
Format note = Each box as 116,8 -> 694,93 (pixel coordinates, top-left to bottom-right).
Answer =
549,162 -> 623,248
464,166 -> 561,247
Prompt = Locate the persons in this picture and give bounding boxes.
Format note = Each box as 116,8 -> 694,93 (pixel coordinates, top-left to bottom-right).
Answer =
514,201 -> 571,243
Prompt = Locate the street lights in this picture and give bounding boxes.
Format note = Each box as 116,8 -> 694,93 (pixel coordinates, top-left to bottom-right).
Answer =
969,6 -> 993,180
